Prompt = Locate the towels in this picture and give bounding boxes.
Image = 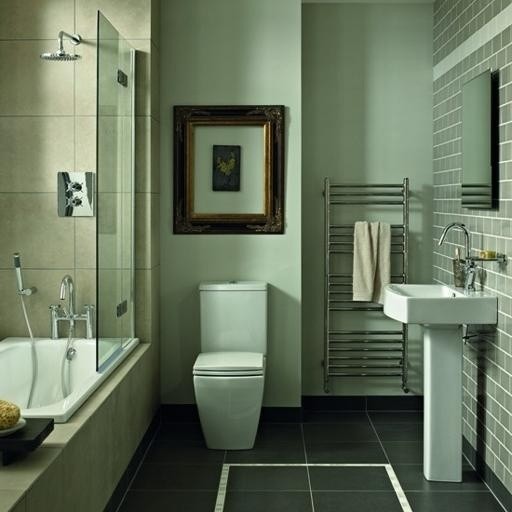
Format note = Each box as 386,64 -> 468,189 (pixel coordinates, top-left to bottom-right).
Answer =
351,220 -> 393,304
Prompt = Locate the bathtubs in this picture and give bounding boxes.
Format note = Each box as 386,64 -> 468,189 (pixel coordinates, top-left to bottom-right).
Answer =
0,336 -> 140,424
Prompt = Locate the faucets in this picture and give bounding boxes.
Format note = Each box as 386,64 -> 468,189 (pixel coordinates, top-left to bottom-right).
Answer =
437,222 -> 475,293
59,276 -> 75,315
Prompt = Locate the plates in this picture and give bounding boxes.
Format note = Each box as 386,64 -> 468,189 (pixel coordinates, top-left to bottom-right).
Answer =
0,418 -> 29,436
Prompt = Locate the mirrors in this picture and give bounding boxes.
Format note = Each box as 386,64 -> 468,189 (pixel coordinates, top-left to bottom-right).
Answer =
459,68 -> 498,211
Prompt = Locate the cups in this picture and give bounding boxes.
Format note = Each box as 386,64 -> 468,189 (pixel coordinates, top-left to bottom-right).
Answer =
453,259 -> 467,287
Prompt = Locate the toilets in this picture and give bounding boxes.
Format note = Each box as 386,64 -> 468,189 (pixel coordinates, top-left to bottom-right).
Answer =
193,280 -> 268,452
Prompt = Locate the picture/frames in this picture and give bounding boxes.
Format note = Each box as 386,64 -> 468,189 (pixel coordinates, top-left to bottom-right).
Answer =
171,103 -> 286,236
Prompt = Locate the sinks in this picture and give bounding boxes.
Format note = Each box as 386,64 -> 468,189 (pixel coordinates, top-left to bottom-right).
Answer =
383,283 -> 498,325
